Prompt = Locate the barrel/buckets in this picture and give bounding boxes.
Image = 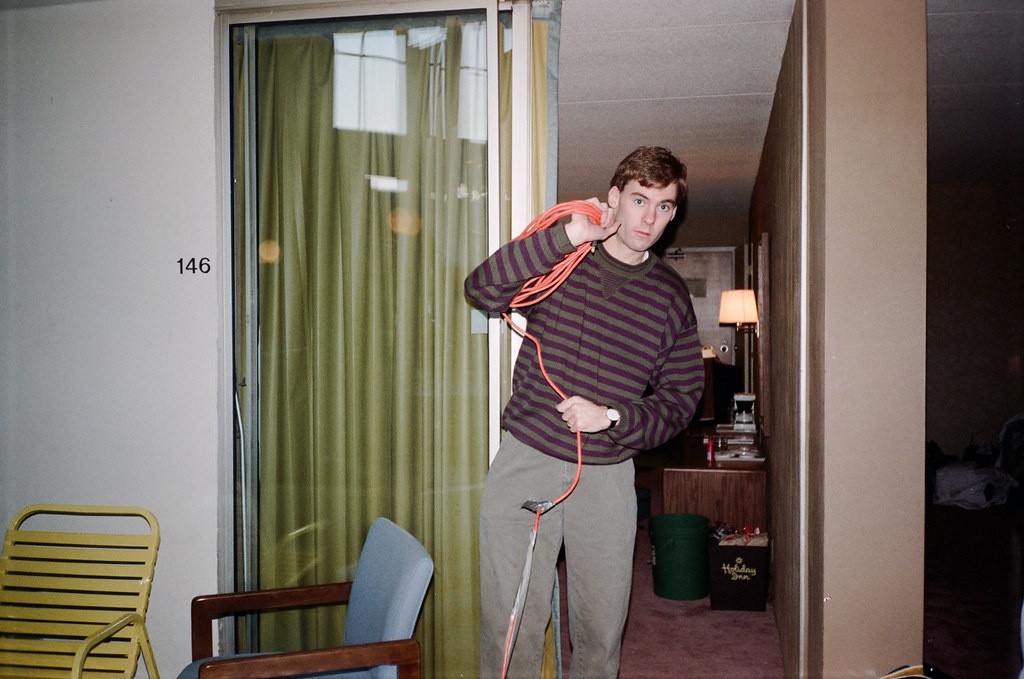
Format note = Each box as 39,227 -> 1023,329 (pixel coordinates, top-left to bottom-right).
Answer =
648,513 -> 713,600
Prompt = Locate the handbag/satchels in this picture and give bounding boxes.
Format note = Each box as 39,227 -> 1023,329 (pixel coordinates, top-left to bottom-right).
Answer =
710,537 -> 772,612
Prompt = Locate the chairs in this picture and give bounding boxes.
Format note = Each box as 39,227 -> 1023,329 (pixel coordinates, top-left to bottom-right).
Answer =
175,518 -> 433,679
0,504 -> 160,679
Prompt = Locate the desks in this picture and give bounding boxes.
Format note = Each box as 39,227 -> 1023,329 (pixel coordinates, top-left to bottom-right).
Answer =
663,446 -> 766,535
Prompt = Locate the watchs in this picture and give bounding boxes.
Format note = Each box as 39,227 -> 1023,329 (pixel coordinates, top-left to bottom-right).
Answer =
606,406 -> 620,429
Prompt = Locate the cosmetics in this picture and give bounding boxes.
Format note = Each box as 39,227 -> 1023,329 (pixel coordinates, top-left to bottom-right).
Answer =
707,435 -> 715,464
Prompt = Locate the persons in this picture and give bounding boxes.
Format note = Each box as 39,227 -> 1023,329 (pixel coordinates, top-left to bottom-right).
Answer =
464,146 -> 705,679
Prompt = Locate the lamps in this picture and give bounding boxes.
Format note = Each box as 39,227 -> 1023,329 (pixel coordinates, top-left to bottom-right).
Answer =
718,288 -> 759,330
